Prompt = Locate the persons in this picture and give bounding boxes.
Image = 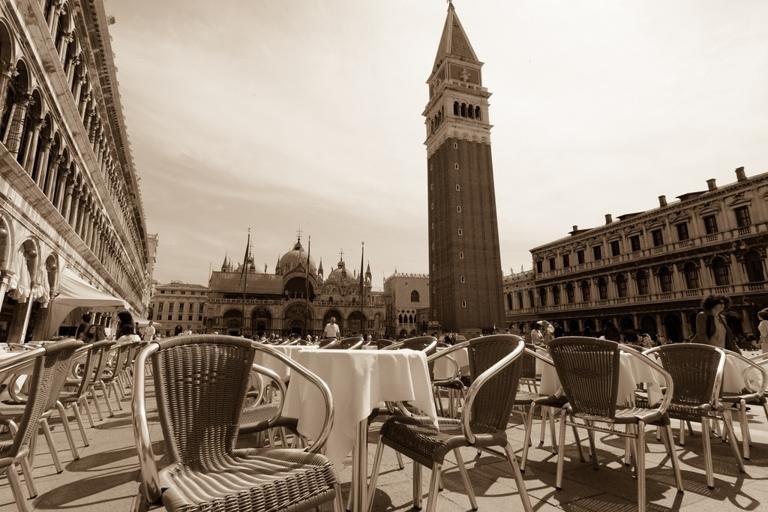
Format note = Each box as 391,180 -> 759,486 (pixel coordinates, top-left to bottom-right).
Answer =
75,310 -> 157,368
530,320 -> 545,347
696,293 -> 743,356
756,309 -> 768,353
174,325 -> 183,336
322,316 -> 341,341
183,324 -> 193,336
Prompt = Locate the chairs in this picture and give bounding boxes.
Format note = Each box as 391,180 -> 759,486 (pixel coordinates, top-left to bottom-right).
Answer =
11,338 -> 152,473
517,323 -> 766,512
131,332 -> 534,512
0,337 -> 88,512
